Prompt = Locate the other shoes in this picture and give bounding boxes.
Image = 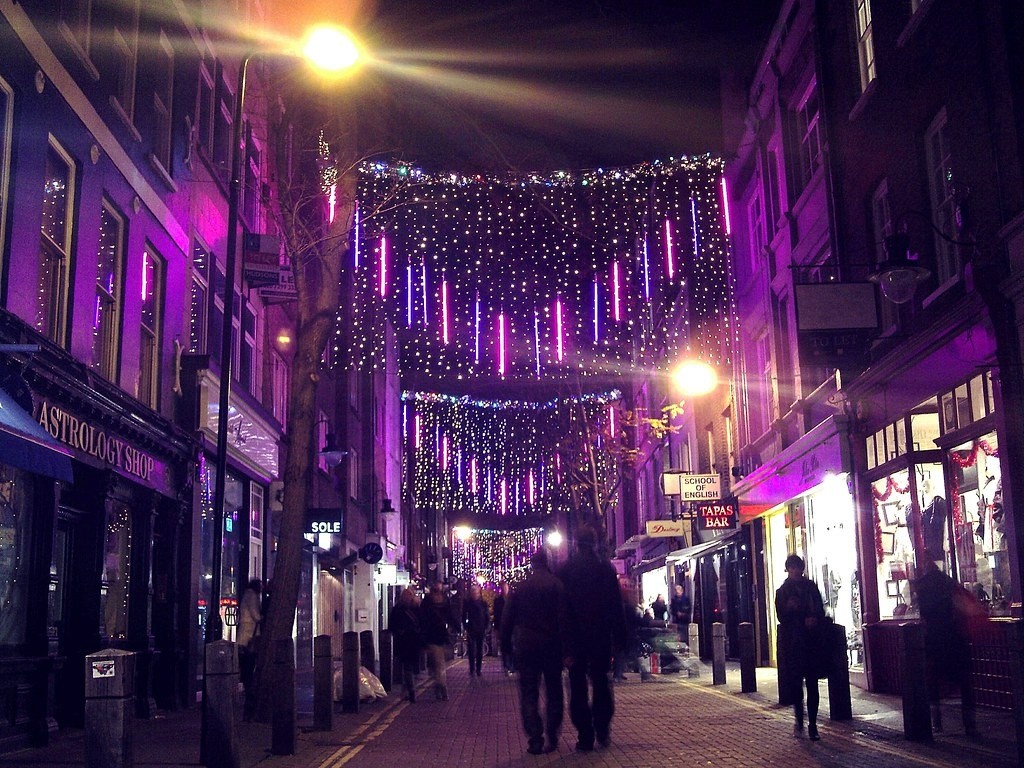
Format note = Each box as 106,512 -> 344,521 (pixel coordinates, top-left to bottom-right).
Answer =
526,741 -> 544,754
442,687 -> 451,701
932,725 -> 942,733
808,724 -> 821,740
597,731 -> 610,745
548,739 -> 559,750
575,740 -> 593,750
794,716 -> 804,738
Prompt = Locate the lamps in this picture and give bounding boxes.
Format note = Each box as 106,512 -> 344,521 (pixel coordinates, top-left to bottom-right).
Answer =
864,166 -> 984,304
313,416 -> 349,468
420,548 -> 437,570
372,489 -> 399,520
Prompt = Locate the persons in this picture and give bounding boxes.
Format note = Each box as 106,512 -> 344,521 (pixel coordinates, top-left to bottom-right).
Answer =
500,555 -> 565,755
235,579 -> 263,725
914,561 -> 977,735
420,598 -> 450,702
670,584 -> 691,642
461,588 -> 490,675
388,589 -> 432,705
492,589 -> 667,679
553,532 -> 627,752
775,555 -> 825,739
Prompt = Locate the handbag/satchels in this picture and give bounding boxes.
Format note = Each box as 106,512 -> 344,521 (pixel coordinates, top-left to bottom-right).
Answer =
247,621 -> 261,656
952,582 -> 993,641
444,626 -> 457,647
